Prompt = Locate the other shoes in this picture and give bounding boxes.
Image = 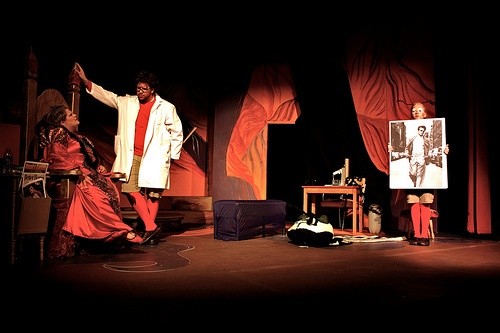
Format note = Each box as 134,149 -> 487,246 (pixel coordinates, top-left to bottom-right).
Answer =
121,233 -> 143,243
419,238 -> 429,246
410,237 -> 419,245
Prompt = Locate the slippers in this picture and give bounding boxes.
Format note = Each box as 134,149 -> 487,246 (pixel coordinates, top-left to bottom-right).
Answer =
140,227 -> 159,244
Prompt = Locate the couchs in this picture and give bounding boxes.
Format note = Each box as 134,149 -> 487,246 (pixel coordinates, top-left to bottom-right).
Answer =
137,196 -> 214,226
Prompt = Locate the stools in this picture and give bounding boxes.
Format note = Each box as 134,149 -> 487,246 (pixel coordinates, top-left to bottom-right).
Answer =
401,208 -> 439,241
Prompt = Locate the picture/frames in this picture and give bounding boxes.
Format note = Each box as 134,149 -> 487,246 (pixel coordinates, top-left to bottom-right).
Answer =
388,118 -> 449,189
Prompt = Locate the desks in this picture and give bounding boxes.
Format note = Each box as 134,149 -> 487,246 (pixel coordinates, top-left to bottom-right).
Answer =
0,165 -> 51,266
302,185 -> 364,236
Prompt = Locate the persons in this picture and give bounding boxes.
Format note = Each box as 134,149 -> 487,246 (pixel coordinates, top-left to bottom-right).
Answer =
40,105 -> 143,260
405,125 -> 430,188
75,62 -> 183,244
388,103 -> 449,246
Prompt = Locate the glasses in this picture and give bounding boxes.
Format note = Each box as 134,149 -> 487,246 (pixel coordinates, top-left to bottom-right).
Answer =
136,87 -> 151,93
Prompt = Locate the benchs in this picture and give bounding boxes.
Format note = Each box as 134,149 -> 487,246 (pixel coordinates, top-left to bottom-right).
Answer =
213,199 -> 286,242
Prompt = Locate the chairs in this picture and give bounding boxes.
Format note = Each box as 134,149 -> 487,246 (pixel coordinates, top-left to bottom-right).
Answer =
20,46 -> 129,260
321,177 -> 366,233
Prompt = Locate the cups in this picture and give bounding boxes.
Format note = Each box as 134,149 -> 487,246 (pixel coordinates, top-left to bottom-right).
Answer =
332,180 -> 339,186
